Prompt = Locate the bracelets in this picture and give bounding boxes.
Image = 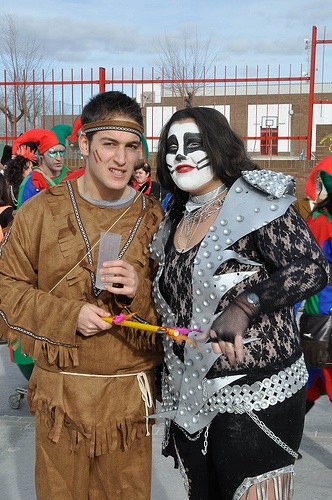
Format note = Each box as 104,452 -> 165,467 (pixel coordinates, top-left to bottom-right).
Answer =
232,298 -> 256,320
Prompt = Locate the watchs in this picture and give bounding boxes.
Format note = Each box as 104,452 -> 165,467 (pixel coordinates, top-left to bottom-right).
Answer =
240,291 -> 261,313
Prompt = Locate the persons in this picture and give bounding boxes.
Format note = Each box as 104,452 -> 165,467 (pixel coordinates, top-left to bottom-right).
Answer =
133,162 -> 160,202
1,91 -> 166,499
127,176 -> 139,191
11,122 -> 75,382
297,181 -> 325,222
294,152 -> 332,459
3,155 -> 33,210
145,107 -> 329,500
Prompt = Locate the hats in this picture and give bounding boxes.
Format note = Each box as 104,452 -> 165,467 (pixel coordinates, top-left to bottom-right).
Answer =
11,124 -> 82,156
305,153 -> 332,203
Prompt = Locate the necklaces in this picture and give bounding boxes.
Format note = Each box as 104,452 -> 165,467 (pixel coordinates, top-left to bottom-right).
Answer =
176,183 -> 228,249
